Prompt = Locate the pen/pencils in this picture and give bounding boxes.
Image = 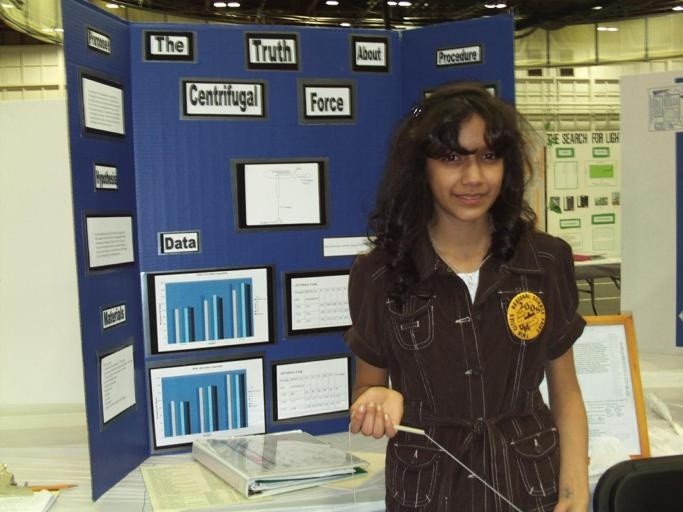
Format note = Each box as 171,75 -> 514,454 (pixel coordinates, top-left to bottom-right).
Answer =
31,484 -> 78,491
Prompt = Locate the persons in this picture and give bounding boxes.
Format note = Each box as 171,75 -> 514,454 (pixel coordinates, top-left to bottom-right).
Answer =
344,84 -> 595,512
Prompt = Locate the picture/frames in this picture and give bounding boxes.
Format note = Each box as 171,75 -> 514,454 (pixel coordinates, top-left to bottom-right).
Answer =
582,313 -> 650,467
